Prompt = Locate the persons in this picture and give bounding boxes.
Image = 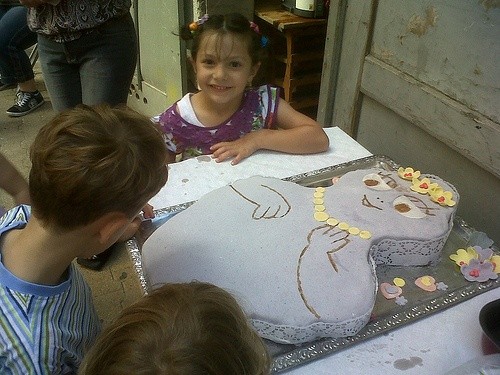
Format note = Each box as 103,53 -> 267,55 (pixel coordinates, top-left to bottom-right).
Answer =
154,13 -> 330,166
0,0 -> 270,375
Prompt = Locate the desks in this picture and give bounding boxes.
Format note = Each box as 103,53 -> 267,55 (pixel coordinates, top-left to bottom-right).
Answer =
254,0 -> 328,110
124,125 -> 500,375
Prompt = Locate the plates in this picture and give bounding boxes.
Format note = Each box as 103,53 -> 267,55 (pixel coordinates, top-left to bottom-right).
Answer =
479,298 -> 500,354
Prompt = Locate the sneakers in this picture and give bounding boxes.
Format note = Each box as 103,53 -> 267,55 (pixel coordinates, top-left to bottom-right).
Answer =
6,88 -> 45,116
0,78 -> 17,90
